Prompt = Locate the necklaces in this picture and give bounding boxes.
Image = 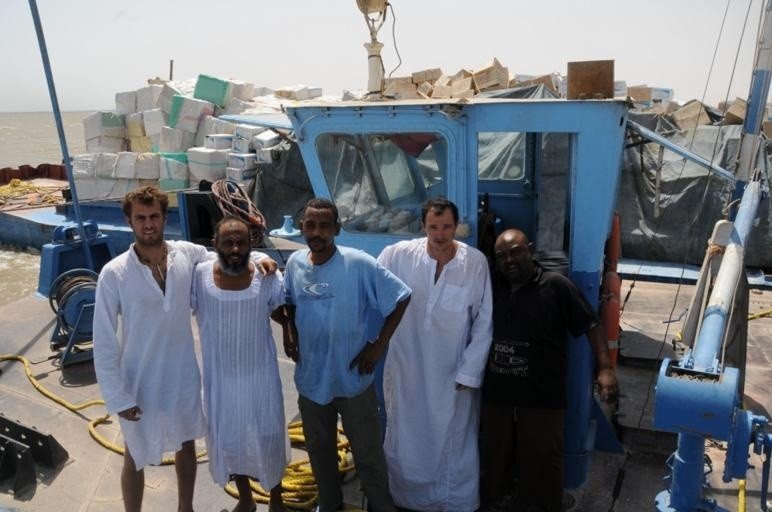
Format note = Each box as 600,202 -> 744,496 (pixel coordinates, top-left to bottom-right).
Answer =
132,244 -> 175,282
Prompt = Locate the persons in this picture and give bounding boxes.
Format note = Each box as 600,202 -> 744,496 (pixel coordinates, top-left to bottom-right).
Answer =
189,215 -> 293,512
285,199 -> 412,512
92,187 -> 278,511
479,231 -> 619,510
378,198 -> 492,512
